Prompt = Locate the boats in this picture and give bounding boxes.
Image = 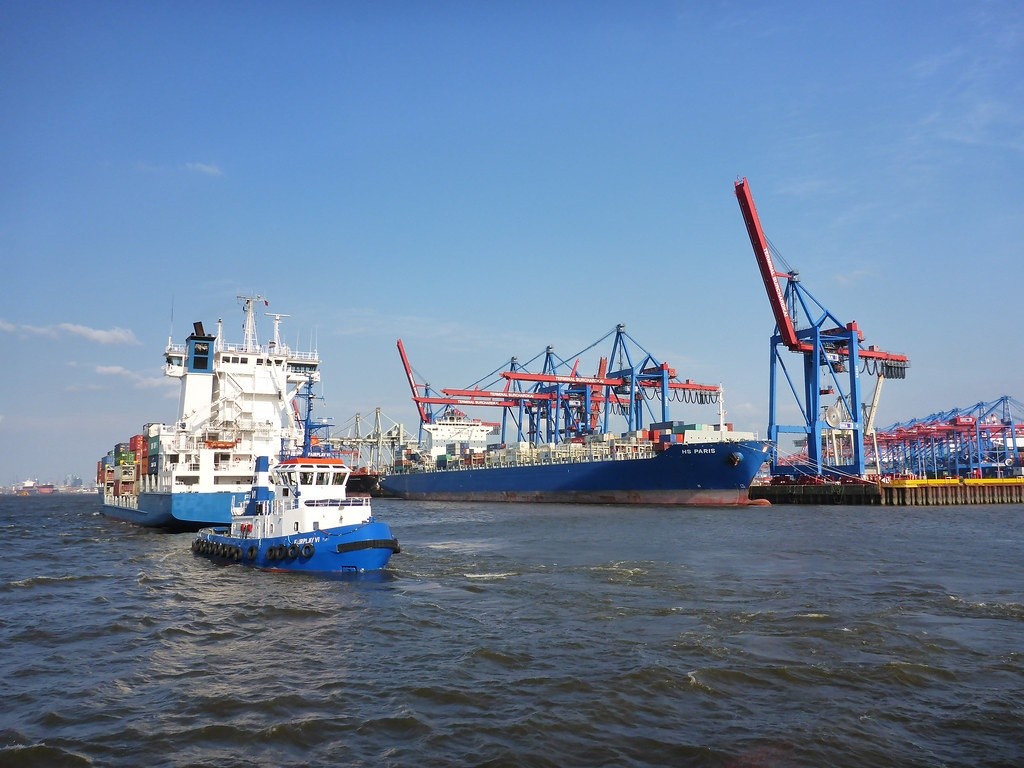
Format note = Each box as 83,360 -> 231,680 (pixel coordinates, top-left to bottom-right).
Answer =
340,467 -> 379,497
192,458 -> 401,576
380,322 -> 776,507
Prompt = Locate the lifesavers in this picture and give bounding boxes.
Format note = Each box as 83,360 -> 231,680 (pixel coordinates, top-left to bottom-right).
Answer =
301,544 -> 314,557
194,539 -> 201,551
266,546 -> 276,560
219,544 -> 228,557
214,543 -> 222,554
247,545 -> 257,560
199,540 -> 206,551
275,544 -> 287,560
208,541 -> 215,553
287,544 -> 299,559
226,545 -> 235,558
233,548 -> 243,562
235,457 -> 241,463
235,437 -> 242,443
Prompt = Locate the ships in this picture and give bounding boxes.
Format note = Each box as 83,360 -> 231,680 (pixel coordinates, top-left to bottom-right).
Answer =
94,293 -> 320,532
